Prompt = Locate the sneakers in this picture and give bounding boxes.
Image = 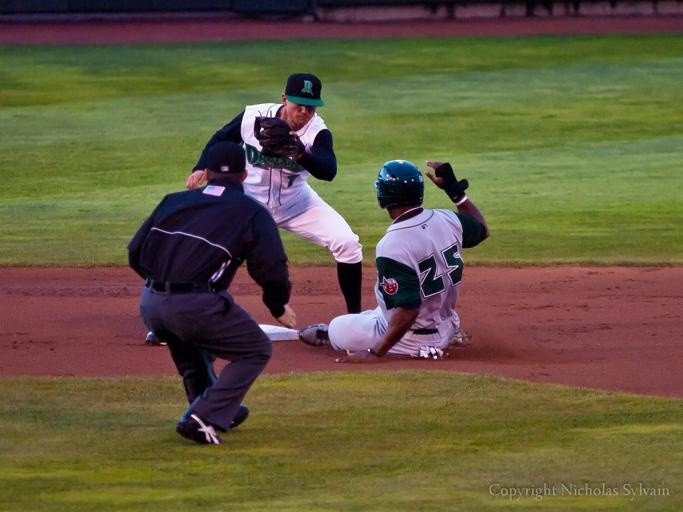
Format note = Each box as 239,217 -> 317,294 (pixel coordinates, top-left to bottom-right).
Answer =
298,323 -> 330,346
177,410 -> 220,444
229,406 -> 249,430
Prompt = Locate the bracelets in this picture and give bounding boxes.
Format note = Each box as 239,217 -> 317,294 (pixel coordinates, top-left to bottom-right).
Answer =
366,347 -> 381,357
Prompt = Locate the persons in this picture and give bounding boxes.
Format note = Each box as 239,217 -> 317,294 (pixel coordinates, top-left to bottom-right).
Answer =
124,140 -> 296,447
142,74 -> 363,348
297,154 -> 491,365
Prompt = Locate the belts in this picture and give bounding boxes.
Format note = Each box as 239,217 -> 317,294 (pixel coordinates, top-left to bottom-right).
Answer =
144,279 -> 207,294
410,327 -> 438,334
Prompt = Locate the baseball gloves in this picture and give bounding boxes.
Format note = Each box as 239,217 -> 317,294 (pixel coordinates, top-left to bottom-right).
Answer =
254,117 -> 306,163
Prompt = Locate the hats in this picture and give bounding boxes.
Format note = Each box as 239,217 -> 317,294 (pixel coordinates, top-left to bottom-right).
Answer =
286,74 -> 323,106
208,143 -> 245,174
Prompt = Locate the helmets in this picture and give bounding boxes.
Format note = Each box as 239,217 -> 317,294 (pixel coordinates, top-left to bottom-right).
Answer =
374,160 -> 423,208
